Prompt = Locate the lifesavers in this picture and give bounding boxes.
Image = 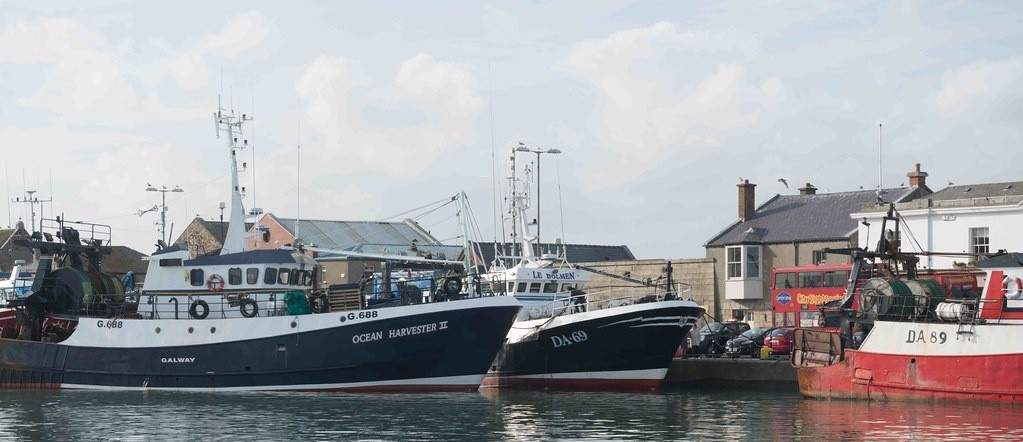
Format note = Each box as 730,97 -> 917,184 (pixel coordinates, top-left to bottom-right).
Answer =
1000,275 -> 1022,299
240,299 -> 258,317
444,277 -> 462,295
208,274 -> 223,291
97,299 -> 114,318
310,294 -> 328,312
191,299 -> 209,319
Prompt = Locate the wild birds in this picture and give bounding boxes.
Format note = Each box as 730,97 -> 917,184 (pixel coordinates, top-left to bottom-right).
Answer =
945,180 -> 955,185
860,186 -> 863,189
777,179 -> 789,189
900,181 -> 904,187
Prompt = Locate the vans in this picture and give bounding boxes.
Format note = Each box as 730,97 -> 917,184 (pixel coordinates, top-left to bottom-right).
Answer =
686,322 -> 751,358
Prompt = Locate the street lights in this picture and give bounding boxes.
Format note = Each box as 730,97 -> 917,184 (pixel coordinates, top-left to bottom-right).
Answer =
145,187 -> 184,244
515,146 -> 561,260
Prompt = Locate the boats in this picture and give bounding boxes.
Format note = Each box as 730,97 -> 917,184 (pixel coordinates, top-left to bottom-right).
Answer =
0,93 -> 526,397
447,145 -> 707,395
790,122 -> 1023,402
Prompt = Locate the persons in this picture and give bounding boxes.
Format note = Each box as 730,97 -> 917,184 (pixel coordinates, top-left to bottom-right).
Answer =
567,286 -> 587,313
784,280 -> 792,288
809,280 -> 814,287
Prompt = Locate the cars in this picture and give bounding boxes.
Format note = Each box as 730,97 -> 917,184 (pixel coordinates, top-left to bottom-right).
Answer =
724,326 -> 778,358
763,327 -> 796,354
